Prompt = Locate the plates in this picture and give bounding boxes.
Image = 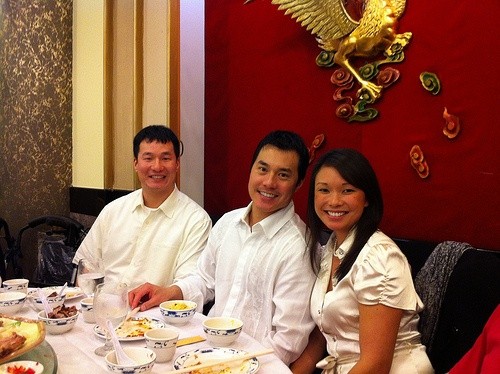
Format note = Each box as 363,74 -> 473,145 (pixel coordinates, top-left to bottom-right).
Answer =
174,347 -> 261,374
93,315 -> 165,341
28,285 -> 84,302
0,313 -> 46,365
0,361 -> 46,374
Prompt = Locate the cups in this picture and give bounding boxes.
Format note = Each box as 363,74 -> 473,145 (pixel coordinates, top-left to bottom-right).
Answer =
80,297 -> 100,323
145,328 -> 180,363
3,278 -> 29,298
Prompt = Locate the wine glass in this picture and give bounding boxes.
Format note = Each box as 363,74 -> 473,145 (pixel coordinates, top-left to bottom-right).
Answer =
92,282 -> 128,358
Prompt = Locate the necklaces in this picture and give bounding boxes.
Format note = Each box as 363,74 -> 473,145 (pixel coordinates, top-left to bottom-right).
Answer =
333,237 -> 344,258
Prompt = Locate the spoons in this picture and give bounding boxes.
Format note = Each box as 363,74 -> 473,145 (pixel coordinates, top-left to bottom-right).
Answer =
105,320 -> 138,367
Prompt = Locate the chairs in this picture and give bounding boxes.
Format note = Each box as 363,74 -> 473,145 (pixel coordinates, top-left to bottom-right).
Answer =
415,236 -> 500,374
0,216 -> 88,287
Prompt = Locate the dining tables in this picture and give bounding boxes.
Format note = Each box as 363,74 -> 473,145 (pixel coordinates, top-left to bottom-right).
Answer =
0,285 -> 295,374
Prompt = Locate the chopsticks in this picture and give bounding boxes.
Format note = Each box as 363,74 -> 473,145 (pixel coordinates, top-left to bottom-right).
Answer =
172,350 -> 273,374
109,304 -> 143,336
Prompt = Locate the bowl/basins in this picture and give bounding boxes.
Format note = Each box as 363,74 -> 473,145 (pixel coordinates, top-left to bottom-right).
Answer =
159,300 -> 198,326
105,345 -> 156,374
0,291 -> 27,314
37,309 -> 80,334
202,317 -> 244,346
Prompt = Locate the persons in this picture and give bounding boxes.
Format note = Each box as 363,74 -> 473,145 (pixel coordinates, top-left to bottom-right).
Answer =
289,147 -> 435,374
72,125 -> 212,308
128,129 -> 321,366
